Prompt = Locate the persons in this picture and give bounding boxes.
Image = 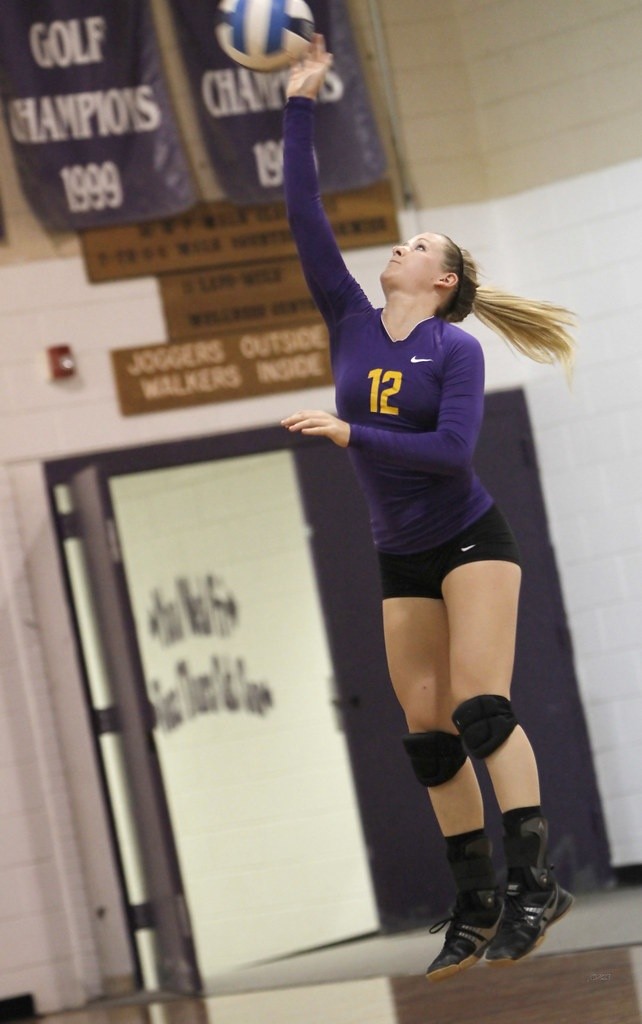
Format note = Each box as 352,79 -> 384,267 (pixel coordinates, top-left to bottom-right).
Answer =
281,32 -> 576,981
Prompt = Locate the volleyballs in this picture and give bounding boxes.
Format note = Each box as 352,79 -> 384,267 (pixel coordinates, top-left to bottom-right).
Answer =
212,0 -> 315,74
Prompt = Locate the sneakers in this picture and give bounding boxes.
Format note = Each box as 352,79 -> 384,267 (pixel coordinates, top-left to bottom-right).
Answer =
426,886 -> 507,979
484,864 -> 573,962
